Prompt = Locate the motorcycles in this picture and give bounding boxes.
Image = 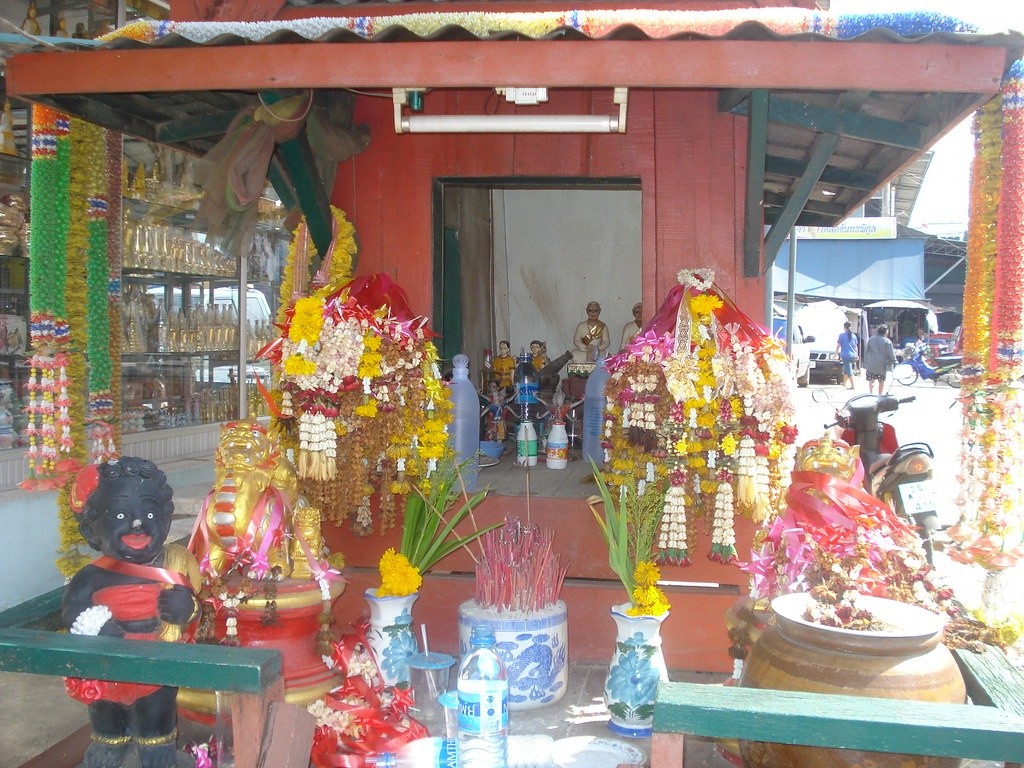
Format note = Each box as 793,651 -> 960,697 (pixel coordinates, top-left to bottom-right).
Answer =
894,341 -> 964,388
811,362 -> 942,572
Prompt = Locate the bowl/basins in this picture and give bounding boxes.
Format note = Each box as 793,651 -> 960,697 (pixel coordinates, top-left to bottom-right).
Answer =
476,440 -> 506,460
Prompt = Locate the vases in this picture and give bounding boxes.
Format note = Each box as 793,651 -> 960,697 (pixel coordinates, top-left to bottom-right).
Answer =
604,603 -> 672,737
364,587 -> 421,691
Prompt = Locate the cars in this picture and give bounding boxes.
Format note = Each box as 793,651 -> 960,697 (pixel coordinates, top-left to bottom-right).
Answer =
809,340 -> 844,386
773,316 -> 816,387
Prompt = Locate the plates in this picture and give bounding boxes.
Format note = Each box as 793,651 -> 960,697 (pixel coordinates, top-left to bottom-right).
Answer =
553,735 -> 648,768
477,460 -> 501,467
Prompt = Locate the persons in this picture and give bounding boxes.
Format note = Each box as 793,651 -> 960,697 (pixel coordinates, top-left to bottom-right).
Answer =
480,301 -> 642,441
862,323 -> 898,396
59,456 -> 203,768
833,322 -> 860,390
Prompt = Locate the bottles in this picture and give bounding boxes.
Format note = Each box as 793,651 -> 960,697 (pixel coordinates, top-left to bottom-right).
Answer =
516,420 -> 537,467
545,422 -> 569,470
444,353 -> 480,492
376,735 -> 554,768
480,350 -> 493,390
513,353 -> 540,435
456,623 -> 509,768
582,357 -> 611,464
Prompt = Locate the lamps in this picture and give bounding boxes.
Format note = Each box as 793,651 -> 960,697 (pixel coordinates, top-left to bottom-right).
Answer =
393,88 -> 629,135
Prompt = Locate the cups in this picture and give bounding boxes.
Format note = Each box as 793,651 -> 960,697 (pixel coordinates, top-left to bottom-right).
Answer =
407,652 -> 456,730
439,691 -> 459,737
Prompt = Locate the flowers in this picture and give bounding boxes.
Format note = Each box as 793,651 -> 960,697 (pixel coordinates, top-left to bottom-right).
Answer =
378,462 -> 502,593
583,453 -> 674,618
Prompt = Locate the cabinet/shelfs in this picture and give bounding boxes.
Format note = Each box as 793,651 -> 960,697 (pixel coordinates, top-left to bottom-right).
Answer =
0,0 -> 292,494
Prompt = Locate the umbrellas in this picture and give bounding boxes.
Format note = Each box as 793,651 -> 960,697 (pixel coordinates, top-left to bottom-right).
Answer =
862,300 -> 940,311
